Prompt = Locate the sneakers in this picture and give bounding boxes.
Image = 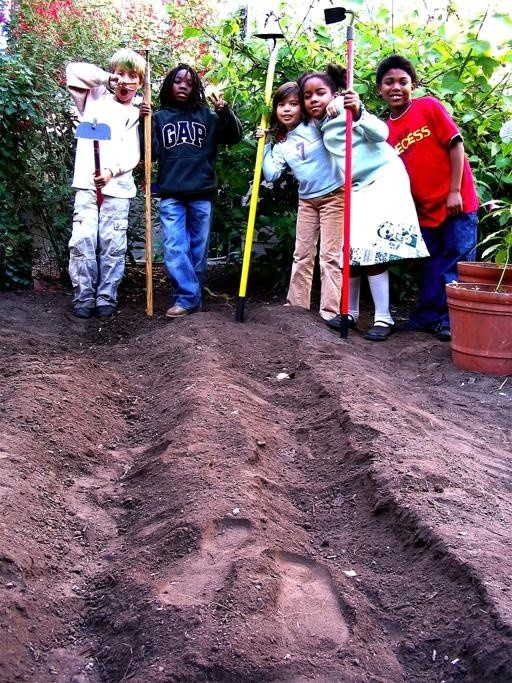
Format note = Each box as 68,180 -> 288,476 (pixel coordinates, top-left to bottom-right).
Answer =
97,305 -> 113,320
77,307 -> 91,319
165,305 -> 202,318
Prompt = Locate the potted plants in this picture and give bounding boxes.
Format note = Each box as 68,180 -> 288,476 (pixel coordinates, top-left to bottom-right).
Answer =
443,278 -> 512,377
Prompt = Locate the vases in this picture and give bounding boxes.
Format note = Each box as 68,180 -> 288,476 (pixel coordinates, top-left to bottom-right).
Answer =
455,260 -> 512,288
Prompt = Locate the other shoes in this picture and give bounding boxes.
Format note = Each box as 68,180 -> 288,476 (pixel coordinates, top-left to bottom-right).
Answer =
393,320 -> 415,332
432,324 -> 452,340
320,310 -> 335,322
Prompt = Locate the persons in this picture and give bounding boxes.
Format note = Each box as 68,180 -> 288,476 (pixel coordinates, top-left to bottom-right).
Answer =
138,61 -> 243,320
254,79 -> 347,329
270,64 -> 431,341
373,54 -> 478,344
63,46 -> 147,319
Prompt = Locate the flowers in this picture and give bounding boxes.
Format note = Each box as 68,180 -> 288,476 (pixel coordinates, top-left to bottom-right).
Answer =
469,197 -> 512,293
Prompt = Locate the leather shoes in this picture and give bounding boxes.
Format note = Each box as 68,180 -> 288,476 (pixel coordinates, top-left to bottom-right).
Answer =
327,315 -> 356,329
365,321 -> 394,340
